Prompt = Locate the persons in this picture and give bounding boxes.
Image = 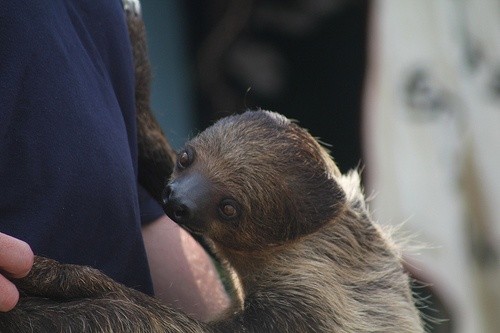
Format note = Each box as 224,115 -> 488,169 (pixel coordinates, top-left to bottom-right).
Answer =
0,0 -> 244,325
